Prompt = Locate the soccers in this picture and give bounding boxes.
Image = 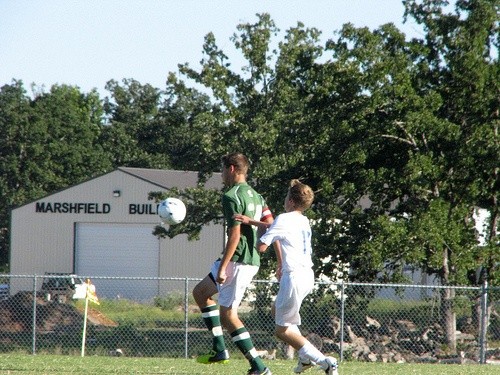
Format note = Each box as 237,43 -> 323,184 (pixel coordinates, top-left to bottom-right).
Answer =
158,197 -> 186,225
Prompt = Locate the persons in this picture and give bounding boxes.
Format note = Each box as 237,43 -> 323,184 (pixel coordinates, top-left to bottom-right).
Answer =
232,179 -> 338,375
193,152 -> 283,375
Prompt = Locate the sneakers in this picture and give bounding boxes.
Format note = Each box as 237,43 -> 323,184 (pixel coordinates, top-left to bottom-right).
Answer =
293,360 -> 316,373
325,355 -> 339,375
195,348 -> 232,364
246,366 -> 272,375
